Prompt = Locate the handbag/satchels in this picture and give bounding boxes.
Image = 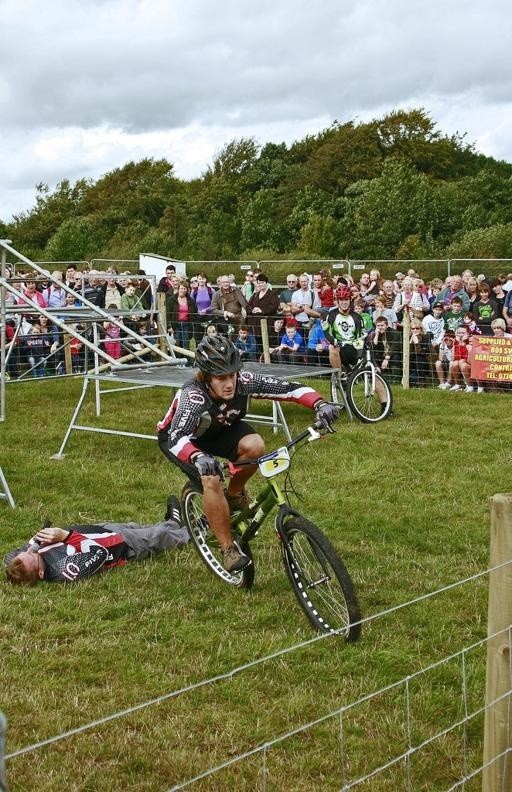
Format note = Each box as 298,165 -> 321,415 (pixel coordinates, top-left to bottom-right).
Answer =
248,293 -> 257,332
421,294 -> 434,317
308,291 -> 314,319
396,293 -> 403,321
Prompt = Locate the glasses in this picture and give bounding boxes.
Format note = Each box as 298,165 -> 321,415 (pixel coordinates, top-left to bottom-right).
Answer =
29,551 -> 39,573
416,285 -> 421,288
190,280 -> 198,284
411,327 -> 419,330
249,275 -> 253,277
75,278 -> 82,280
107,271 -> 117,274
288,281 -> 295,283
397,279 -> 404,281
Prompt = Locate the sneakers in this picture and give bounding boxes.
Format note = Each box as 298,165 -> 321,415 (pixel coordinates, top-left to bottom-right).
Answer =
32,369 -> 37,378
224,488 -> 255,512
192,512 -> 210,539
220,544 -> 250,573
438,383 -> 452,390
5,372 -> 10,380
477,387 -> 484,394
464,385 -> 473,393
449,384 -> 462,391
164,495 -> 182,528
39,369 -> 45,376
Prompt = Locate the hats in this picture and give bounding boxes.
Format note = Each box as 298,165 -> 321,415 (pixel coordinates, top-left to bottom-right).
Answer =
36,270 -> 53,279
287,318 -> 298,326
433,302 -> 443,309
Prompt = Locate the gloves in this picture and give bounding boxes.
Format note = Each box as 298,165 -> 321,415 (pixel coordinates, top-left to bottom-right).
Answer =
192,454 -> 226,483
317,403 -> 340,434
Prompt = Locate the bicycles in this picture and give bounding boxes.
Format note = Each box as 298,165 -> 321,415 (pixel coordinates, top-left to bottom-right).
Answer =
331,328 -> 393,423
181,405 -> 361,644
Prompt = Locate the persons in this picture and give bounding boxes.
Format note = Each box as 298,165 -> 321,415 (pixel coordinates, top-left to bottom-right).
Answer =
3,261 -> 347,376
323,286 -> 402,420
158,336 -> 339,573
3,495 -> 215,585
348,268 -> 512,393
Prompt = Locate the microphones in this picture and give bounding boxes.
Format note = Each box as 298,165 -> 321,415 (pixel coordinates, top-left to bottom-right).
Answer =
30,520 -> 52,553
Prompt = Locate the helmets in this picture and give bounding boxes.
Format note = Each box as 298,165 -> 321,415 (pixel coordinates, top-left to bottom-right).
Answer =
336,286 -> 354,311
193,336 -> 243,393
